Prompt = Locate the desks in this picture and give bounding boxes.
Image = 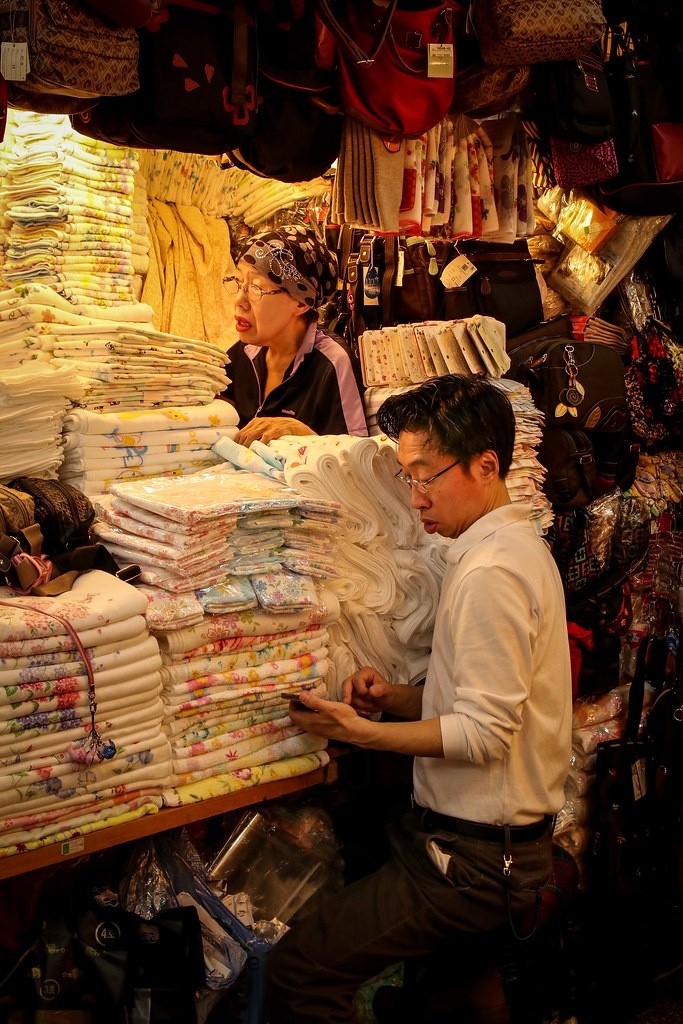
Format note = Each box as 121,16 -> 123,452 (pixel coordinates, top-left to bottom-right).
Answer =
0,759 -> 338,882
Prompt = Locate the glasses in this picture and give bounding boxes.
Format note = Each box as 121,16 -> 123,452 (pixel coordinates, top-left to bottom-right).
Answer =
394,459 -> 462,493
222,276 -> 285,301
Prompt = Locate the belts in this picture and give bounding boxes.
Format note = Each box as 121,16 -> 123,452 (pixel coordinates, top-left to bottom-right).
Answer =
408,794 -> 553,843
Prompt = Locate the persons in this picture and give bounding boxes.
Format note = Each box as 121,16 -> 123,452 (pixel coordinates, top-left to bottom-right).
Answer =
281,369 -> 573,1024
213,225 -> 370,450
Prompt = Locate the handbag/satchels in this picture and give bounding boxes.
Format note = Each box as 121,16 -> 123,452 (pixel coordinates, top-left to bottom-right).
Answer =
0,484 -> 81,596
596,635 -> 683,833
7,477 -> 142,584
326,226 -> 641,597
0,0 -> 683,217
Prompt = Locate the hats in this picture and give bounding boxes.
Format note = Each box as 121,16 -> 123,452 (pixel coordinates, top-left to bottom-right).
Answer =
242,225 -> 339,309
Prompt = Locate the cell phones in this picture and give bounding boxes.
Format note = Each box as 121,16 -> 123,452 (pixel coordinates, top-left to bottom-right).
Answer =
281,693 -> 316,713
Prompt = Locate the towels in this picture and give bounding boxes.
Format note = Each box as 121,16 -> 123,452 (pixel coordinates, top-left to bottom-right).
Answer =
0,109 -> 681,862
